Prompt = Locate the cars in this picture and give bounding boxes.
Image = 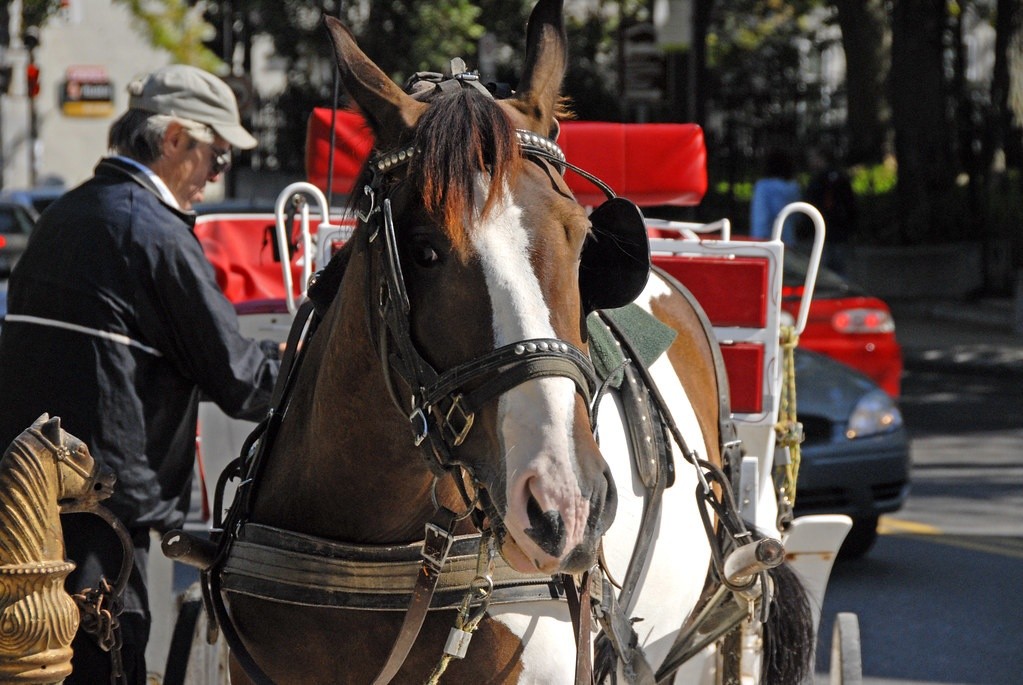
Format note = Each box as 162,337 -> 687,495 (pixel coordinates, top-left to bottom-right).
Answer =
793,346 -> 911,567
0,188 -> 65,272
775,251 -> 901,401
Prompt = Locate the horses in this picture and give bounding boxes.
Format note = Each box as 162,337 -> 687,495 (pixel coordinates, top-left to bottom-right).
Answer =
219,1 -> 724,685
0,411 -> 117,565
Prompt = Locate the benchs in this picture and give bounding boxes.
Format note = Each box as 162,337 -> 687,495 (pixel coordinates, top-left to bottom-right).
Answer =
194,112 -> 785,421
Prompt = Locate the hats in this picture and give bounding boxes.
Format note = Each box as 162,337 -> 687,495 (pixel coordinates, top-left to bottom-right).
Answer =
128,65 -> 258,150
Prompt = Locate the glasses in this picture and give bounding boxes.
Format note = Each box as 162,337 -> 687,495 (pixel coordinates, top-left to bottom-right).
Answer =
203,140 -> 232,176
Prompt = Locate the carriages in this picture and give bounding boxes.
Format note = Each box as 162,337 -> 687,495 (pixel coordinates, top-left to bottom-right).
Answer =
163,0 -> 863,685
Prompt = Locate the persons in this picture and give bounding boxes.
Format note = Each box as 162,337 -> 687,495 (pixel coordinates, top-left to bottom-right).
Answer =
305,89 -> 375,209
0,65 -> 304,685
276,63 -> 325,167
751,147 -> 802,252
